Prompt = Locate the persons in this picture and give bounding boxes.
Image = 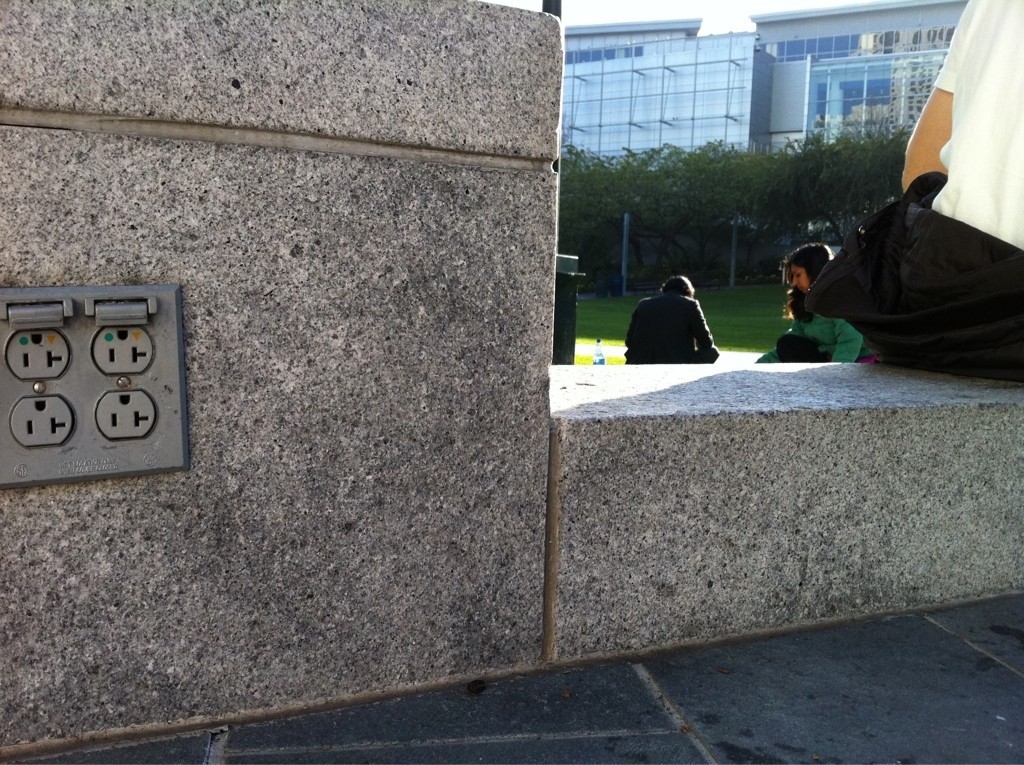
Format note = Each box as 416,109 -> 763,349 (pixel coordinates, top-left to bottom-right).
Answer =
755,242 -> 876,363
901,0 -> 1024,252
624,276 -> 719,364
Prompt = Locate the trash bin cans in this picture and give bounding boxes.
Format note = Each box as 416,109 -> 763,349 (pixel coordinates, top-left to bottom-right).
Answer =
553,255 -> 587,364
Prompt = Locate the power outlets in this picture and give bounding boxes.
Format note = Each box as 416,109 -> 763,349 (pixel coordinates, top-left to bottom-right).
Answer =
0,284 -> 190,488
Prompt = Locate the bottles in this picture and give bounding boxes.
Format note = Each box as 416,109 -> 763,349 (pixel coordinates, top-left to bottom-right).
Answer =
592,339 -> 605,365
